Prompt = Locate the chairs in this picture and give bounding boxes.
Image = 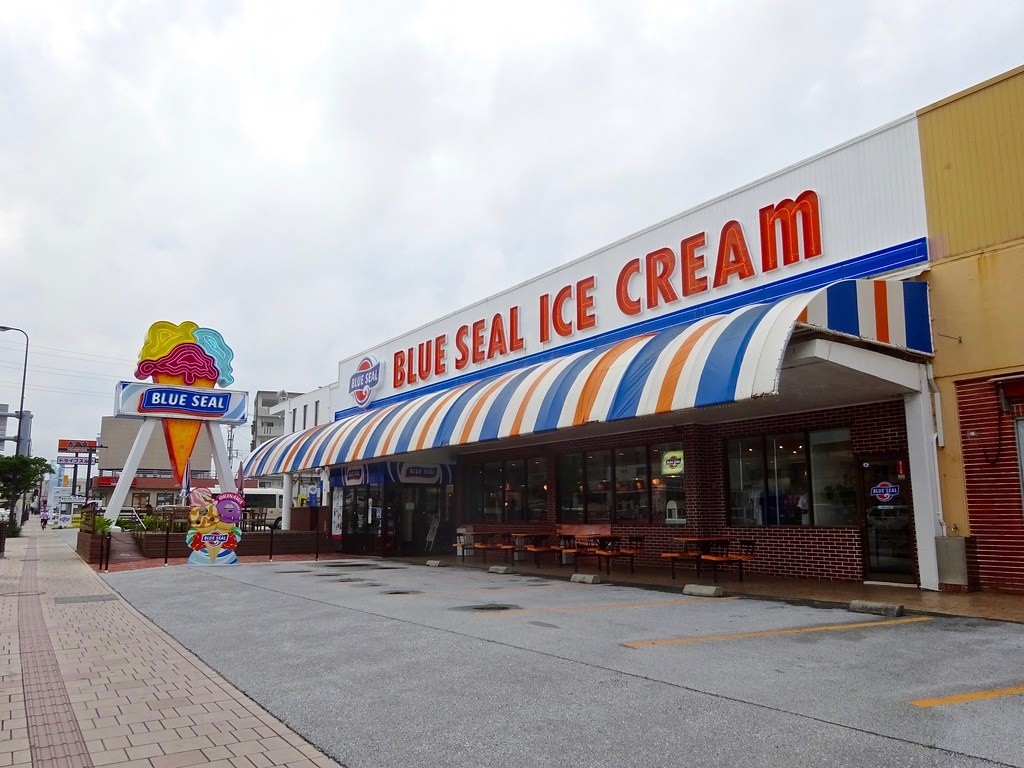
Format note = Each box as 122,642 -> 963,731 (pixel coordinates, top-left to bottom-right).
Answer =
452,530 -> 756,583
154,504 -> 268,533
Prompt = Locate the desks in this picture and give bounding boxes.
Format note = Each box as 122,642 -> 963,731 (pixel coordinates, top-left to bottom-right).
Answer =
456,532 -> 503,545
574,534 -> 629,550
672,536 -> 732,555
510,531 -> 558,547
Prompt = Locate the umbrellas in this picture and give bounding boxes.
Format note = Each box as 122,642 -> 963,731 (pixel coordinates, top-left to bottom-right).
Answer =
178,457 -> 192,507
236,460 -> 246,500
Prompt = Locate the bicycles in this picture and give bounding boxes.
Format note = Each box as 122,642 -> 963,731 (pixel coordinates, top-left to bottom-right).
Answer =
40,517 -> 50,530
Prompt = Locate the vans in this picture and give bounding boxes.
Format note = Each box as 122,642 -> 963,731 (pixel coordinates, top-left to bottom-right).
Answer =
180,487 -> 296,530
867,505 -> 909,532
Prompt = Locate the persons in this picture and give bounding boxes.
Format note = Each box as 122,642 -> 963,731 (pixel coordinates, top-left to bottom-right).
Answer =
146,504 -> 159,520
795,483 -> 809,525
39,509 -> 49,531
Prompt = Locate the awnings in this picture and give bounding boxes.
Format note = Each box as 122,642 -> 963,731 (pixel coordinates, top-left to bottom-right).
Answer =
233,274 -> 940,480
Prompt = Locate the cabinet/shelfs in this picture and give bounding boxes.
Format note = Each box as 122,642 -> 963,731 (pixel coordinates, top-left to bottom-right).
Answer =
731,507 -> 753,525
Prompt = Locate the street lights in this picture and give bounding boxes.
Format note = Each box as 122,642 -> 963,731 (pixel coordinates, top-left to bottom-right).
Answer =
0,325 -> 30,527
